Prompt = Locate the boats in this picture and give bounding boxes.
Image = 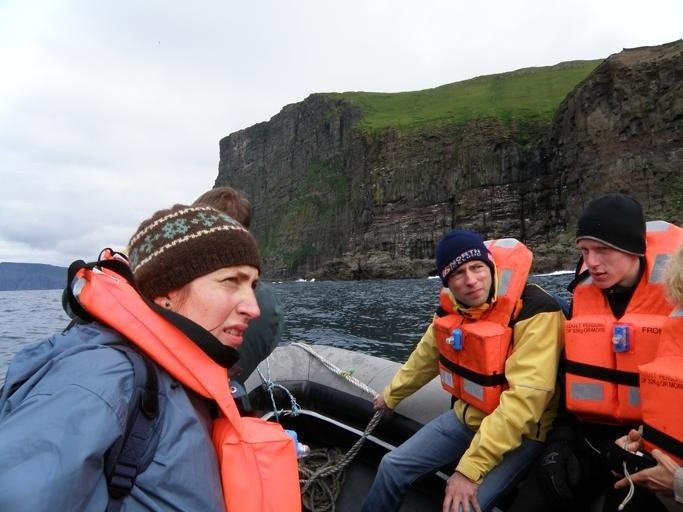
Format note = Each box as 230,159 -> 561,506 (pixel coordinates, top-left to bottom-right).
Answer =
244,343 -> 460,507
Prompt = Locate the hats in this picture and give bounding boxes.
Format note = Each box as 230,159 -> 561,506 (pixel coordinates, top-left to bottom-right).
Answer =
127,203 -> 259,301
575,194 -> 647,257
435,230 -> 494,287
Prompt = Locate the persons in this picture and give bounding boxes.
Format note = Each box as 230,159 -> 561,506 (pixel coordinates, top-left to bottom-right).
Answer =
177,188 -> 281,396
0,202 -> 264,511
612,247 -> 683,505
551,193 -> 683,510
353,228 -> 567,512
